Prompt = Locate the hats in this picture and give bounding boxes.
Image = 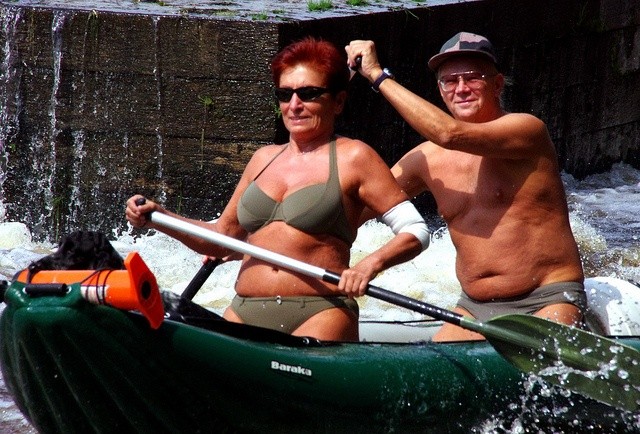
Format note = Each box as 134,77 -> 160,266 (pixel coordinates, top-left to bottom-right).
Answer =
428,32 -> 498,71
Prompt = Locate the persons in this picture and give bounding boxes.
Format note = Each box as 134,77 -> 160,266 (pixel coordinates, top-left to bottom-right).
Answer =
122,38 -> 430,339
341,28 -> 588,341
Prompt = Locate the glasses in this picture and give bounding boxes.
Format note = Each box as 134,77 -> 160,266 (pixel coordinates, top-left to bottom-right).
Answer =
439,70 -> 491,91
273,87 -> 328,101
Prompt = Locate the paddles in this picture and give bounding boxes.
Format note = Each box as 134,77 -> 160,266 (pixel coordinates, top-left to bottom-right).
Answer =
137,198 -> 640,413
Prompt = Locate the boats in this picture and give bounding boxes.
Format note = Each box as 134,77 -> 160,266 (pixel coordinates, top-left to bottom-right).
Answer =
1,230 -> 639,433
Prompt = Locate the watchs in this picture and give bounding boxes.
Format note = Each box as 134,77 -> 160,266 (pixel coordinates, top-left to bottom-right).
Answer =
372,68 -> 396,92
